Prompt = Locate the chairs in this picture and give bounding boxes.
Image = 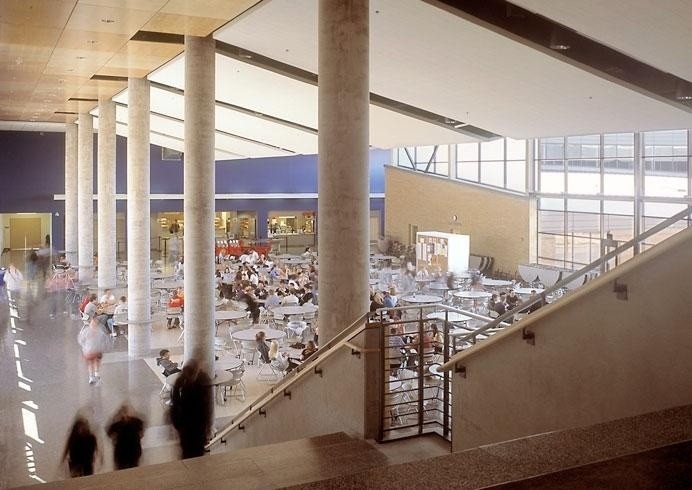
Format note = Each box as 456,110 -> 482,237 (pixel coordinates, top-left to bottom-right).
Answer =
150,261 -> 182,328
116,258 -> 128,283
260,254 -> 318,297
160,369 -> 182,406
215,297 -> 317,405
370,256 -> 549,352
386,363 -> 452,426
115,310 -> 128,338
